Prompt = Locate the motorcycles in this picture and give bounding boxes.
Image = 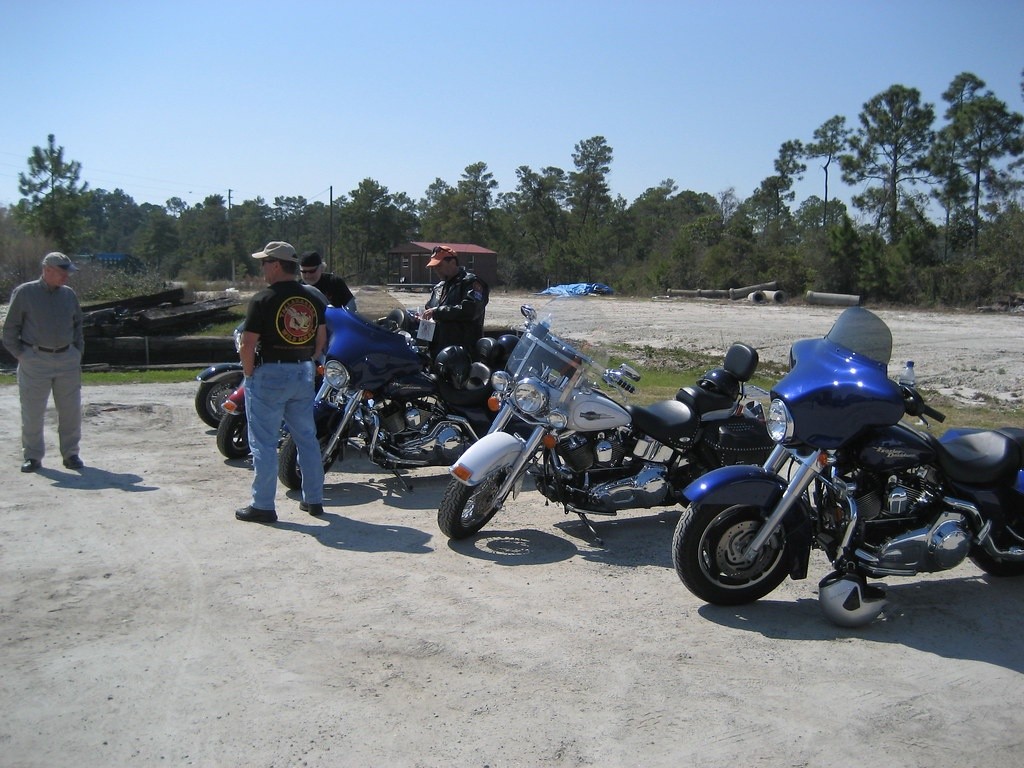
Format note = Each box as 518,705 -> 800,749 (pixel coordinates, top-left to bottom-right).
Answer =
192,308 -> 428,460
669,305 -> 1024,607
271,285 -> 581,489
436,295 -> 783,537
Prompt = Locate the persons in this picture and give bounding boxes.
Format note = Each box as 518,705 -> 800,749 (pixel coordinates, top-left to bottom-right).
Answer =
2,252 -> 85,472
299,250 -> 357,368
236,242 -> 328,524
421,247 -> 489,360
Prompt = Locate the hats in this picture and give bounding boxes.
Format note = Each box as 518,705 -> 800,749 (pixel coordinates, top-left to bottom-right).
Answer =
300,250 -> 321,267
42,252 -> 80,272
251,241 -> 299,263
426,246 -> 457,269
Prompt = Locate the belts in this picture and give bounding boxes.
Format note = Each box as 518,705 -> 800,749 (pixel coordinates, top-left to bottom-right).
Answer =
21,339 -> 70,353
257,358 -> 312,364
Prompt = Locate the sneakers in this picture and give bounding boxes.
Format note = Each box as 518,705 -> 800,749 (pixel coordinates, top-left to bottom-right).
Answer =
21,459 -> 41,472
63,455 -> 83,469
236,505 -> 277,523
300,500 -> 323,515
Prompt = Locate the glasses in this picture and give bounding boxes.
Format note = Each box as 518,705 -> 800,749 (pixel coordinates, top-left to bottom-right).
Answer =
433,246 -> 451,253
301,267 -> 317,274
261,259 -> 276,265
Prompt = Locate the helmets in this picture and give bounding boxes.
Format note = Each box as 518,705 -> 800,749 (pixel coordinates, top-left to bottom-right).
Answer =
818,568 -> 892,629
434,345 -> 472,389
695,368 -> 740,403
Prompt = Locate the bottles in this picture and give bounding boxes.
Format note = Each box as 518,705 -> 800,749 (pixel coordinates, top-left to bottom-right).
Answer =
899,361 -> 915,389
